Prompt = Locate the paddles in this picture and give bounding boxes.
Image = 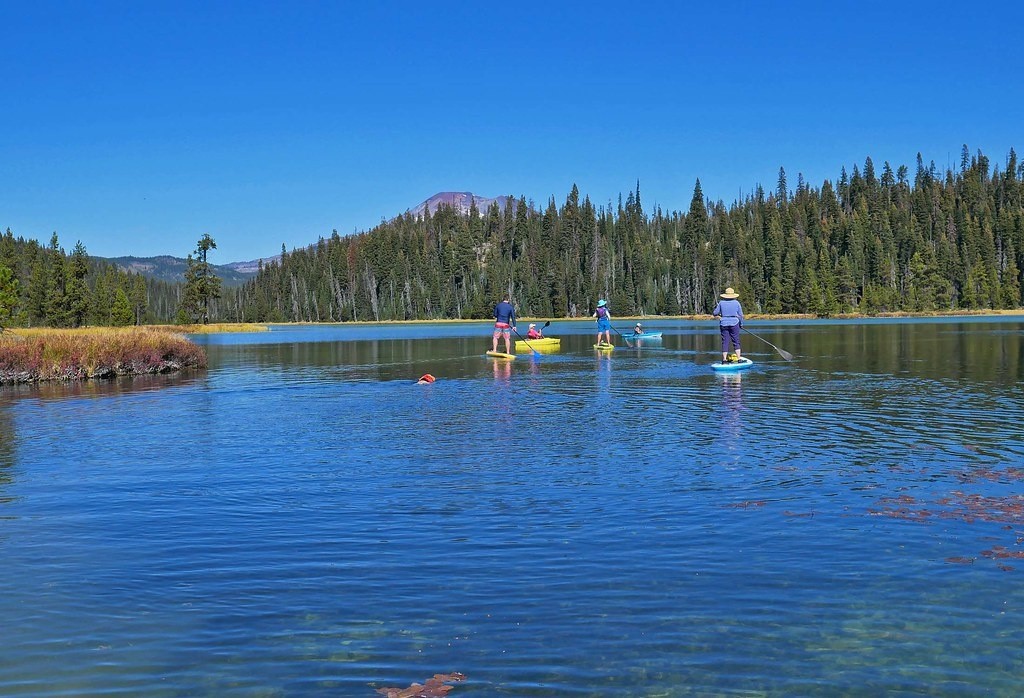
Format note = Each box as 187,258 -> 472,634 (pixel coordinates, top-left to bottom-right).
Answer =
508,324 -> 543,358
609,323 -> 634,348
739,325 -> 794,361
538,320 -> 550,334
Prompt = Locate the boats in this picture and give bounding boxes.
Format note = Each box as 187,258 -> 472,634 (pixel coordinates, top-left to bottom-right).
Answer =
515,344 -> 561,353
514,336 -> 561,346
621,331 -> 663,338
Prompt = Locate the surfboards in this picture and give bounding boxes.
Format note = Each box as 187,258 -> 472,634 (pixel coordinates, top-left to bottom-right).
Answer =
485,348 -> 517,358
593,342 -> 614,350
710,353 -> 754,371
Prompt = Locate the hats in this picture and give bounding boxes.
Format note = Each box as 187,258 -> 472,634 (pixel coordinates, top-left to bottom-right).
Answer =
720,288 -> 739,298
529,323 -> 536,329
637,323 -> 641,327
597,299 -> 607,306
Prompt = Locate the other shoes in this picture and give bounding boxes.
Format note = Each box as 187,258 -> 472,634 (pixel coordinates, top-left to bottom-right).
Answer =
722,360 -> 731,364
738,359 -> 747,363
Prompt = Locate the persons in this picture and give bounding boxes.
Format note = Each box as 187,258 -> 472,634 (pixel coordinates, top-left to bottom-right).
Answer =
634,322 -> 643,334
489,294 -> 517,354
593,300 -> 612,347
527,323 -> 544,339
712,288 -> 748,364
